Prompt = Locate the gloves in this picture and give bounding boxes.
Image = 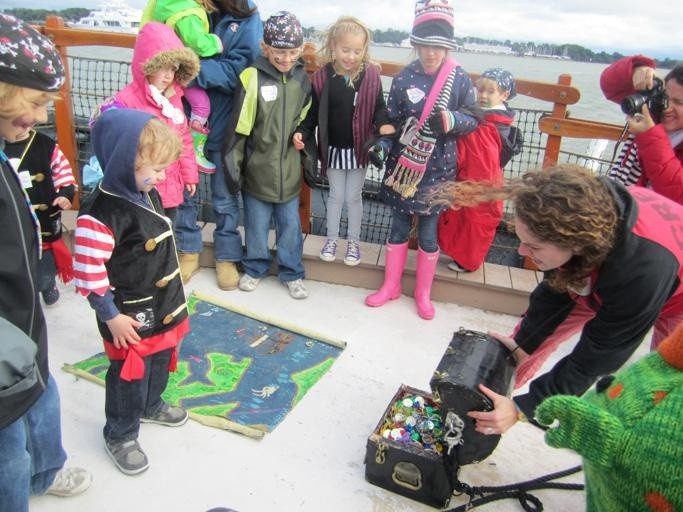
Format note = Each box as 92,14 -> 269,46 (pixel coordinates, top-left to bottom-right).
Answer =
367,138 -> 394,170
426,107 -> 455,137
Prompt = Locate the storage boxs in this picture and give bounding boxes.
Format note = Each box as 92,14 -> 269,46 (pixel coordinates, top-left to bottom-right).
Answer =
363,326 -> 517,510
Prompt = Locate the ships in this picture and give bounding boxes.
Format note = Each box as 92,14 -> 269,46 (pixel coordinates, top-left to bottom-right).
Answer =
67,2 -> 143,35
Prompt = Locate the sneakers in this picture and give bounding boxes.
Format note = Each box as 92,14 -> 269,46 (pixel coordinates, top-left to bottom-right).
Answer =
449,260 -> 467,272
178,252 -> 200,283
42,284 -> 60,307
343,239 -> 361,265
45,466 -> 92,500
320,239 -> 338,263
140,400 -> 189,429
283,277 -> 310,300
240,271 -> 267,295
213,259 -> 239,291
102,436 -> 149,476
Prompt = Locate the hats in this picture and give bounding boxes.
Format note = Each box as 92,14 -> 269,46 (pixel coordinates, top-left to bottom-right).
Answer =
265,9 -> 304,49
411,0 -> 459,49
0,13 -> 67,91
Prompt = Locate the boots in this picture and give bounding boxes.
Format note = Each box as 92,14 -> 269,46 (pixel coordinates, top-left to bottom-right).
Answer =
414,242 -> 442,321
187,122 -> 221,177
365,238 -> 410,312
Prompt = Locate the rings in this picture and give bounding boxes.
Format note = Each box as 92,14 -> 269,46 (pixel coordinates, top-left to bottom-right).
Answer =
488,426 -> 493,433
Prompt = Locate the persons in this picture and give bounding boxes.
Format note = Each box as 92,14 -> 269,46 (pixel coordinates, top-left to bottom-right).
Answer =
86,21 -> 200,234
172,1 -> 265,290
0,10 -> 92,511
1,128 -> 79,308
138,0 -> 223,175
413,160 -> 683,436
446,69 -> 517,274
222,9 -> 313,299
365,0 -> 478,321
599,53 -> 683,202
72,108 -> 191,477
292,15 -> 396,266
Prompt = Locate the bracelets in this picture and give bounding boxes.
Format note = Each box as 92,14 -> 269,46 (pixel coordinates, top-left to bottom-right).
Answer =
513,401 -> 528,422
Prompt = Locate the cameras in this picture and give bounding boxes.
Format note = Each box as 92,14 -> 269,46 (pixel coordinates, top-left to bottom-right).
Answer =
621,77 -> 669,115
